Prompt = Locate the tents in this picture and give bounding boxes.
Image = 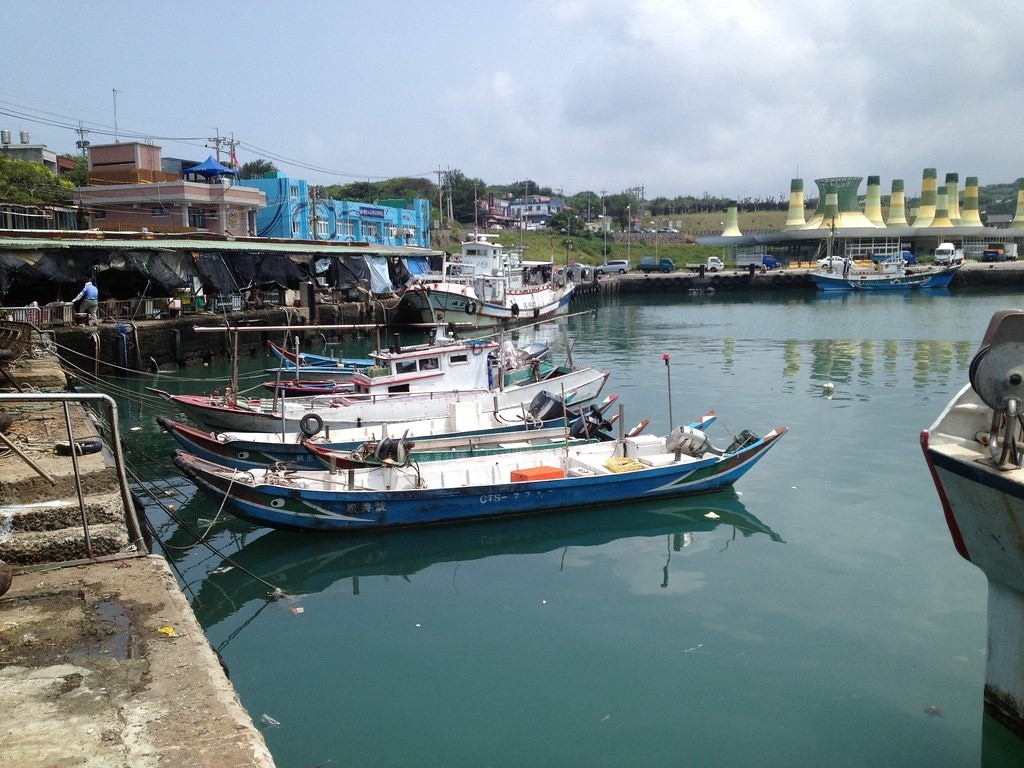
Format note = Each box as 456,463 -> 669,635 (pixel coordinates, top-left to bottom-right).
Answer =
184,154 -> 241,184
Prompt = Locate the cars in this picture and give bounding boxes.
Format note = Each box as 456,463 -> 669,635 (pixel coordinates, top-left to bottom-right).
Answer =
527,223 -> 546,231
817,256 -> 856,269
490,224 -> 503,229
557,263 -> 594,275
627,227 -> 680,234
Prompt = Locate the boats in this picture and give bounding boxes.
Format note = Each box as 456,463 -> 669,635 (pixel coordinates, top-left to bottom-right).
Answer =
301,410 -> 728,470
261,307 -> 597,396
172,354 -> 789,530
154,392 -> 650,470
402,232 -> 576,337
146,364 -> 610,435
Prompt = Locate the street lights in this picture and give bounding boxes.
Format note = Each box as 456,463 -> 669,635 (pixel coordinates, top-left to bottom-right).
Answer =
650,214 -> 658,264
566,215 -> 578,267
598,206 -> 606,263
826,213 -> 840,271
720,219 -> 725,272
627,203 -> 631,270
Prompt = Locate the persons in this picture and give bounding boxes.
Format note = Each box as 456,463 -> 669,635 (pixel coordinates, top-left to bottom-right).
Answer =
71,280 -> 101,327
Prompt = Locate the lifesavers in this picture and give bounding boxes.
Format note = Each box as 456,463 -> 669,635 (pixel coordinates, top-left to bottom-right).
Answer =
301,414 -> 323,435
595,269 -> 599,277
56,440 -> 103,452
575,285 -> 601,295
465,302 -> 476,315
534,309 -> 540,319
513,304 -> 520,315
641,273 -> 812,287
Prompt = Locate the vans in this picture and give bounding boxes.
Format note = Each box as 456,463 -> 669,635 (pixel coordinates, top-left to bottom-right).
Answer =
596,260 -> 632,274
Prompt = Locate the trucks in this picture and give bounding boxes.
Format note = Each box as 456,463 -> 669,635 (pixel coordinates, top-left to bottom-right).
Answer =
637,258 -> 676,273
872,250 -> 915,267
736,254 -> 777,271
933,242 -> 964,266
982,242 -> 1018,262
686,256 -> 725,272
